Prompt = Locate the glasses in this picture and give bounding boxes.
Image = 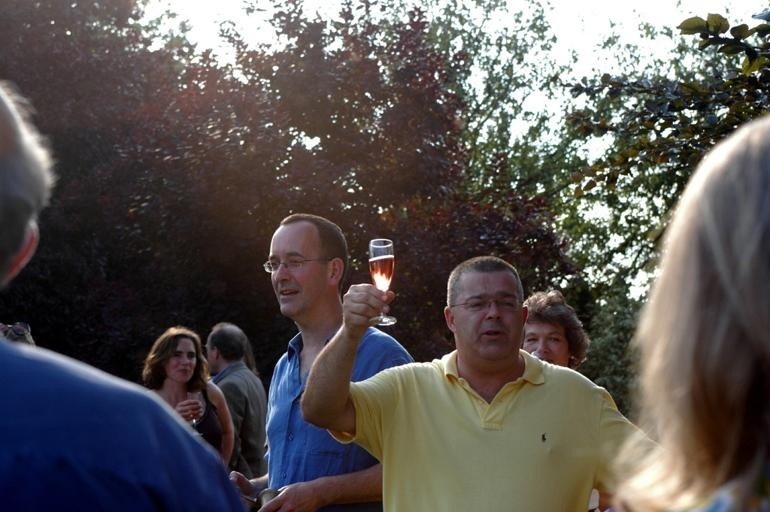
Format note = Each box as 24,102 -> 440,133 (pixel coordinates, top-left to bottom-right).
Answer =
263,258 -> 333,273
446,297 -> 521,312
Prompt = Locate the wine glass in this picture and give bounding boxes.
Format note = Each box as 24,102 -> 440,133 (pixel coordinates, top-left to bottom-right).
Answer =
367,238 -> 398,325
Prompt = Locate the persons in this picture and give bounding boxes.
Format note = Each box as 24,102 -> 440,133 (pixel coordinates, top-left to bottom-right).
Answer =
521,290 -> 590,370
0,78 -> 247,510
298,254 -> 696,511
625,117 -> 770,512
139,325 -> 236,472
221,213 -> 412,511
204,319 -> 268,477
